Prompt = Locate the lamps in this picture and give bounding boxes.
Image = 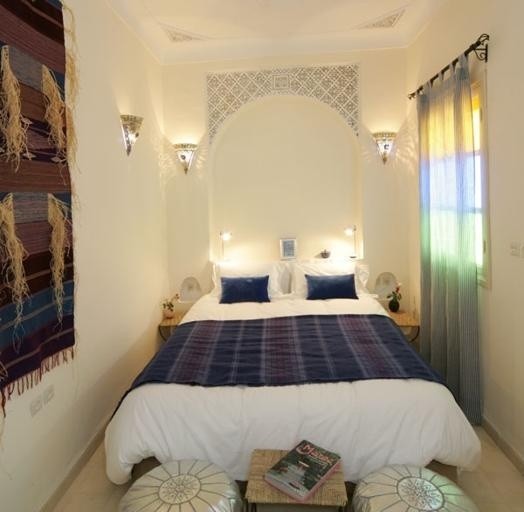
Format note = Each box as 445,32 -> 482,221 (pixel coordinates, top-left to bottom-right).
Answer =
120,114 -> 144,157
374,131 -> 397,164
217,232 -> 232,259
174,143 -> 198,175
344,225 -> 357,258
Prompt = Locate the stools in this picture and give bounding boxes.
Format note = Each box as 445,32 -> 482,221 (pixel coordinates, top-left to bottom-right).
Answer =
115,459 -> 241,512
352,465 -> 483,512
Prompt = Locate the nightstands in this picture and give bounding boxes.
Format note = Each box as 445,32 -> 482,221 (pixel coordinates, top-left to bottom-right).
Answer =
159,311 -> 183,343
385,308 -> 419,343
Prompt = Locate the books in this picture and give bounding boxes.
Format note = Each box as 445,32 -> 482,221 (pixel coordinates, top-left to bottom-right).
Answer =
262,440 -> 342,501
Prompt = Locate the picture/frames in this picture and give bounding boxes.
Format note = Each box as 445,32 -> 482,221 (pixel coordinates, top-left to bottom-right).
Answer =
278,238 -> 298,260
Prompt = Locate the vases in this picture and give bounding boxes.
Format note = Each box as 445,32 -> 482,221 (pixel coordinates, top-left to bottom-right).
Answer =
389,299 -> 400,313
162,308 -> 176,319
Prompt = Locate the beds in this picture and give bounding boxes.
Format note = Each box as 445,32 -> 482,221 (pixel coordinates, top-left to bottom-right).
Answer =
103,258 -> 483,491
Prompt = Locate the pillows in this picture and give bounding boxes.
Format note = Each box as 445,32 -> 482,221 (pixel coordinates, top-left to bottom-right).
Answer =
292,261 -> 364,298
211,260 -> 290,299
219,275 -> 272,303
304,273 -> 360,300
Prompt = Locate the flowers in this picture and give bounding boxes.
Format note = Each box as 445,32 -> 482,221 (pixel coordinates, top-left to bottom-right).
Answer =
386,279 -> 403,302
159,290 -> 184,312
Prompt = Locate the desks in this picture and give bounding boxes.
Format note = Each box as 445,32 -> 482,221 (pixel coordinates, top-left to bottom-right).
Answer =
241,448 -> 348,511
